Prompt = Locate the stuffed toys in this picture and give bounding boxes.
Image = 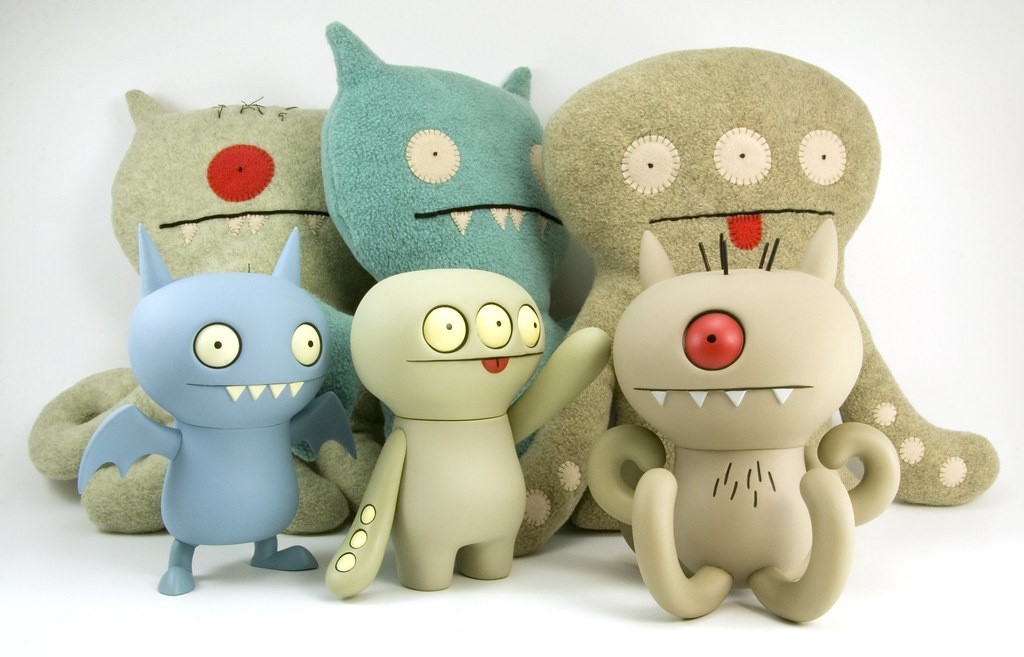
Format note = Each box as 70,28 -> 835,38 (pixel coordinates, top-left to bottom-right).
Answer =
27,89 -> 385,536
291,21 -> 566,462
513,47 -> 1000,557
